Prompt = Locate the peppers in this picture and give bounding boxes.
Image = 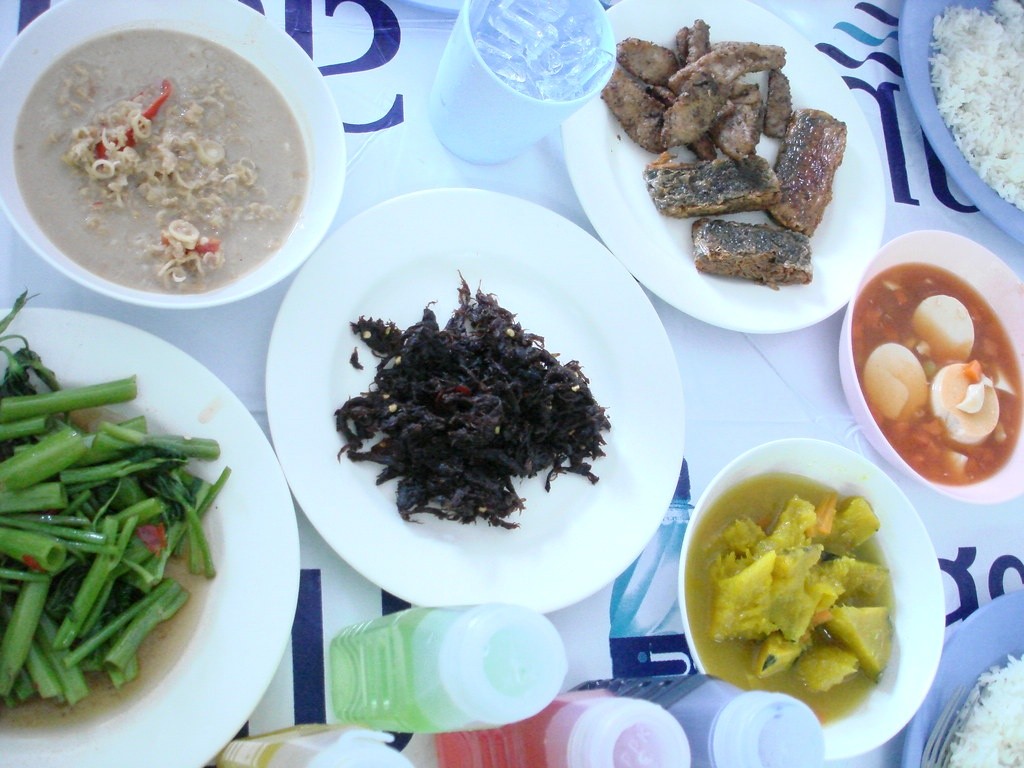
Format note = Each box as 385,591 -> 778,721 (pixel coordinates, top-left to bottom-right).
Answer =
160,235 -> 220,256
96,78 -> 172,162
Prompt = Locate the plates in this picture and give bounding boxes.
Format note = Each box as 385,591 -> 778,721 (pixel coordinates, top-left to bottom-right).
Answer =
263,183 -> 686,614
0,306 -> 302,768
562,0 -> 888,335
896,0 -> 1024,244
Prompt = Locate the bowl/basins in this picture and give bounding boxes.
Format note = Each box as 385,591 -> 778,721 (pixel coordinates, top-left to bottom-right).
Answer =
839,226 -> 1024,505
899,586 -> 1024,768
0,0 -> 349,310
676,435 -> 946,762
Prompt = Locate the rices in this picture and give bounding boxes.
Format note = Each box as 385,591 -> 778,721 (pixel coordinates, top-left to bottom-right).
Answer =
929,0 -> 1024,213
947,649 -> 1024,768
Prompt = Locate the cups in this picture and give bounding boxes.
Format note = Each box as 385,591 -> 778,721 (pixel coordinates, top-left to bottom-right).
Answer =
423,0 -> 618,165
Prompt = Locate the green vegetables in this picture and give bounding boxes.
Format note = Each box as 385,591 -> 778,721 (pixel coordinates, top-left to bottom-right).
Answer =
0,289 -> 231,709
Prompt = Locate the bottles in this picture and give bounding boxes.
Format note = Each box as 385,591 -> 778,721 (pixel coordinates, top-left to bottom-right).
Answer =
215,723 -> 415,768
328,603 -> 569,735
436,689 -> 691,768
568,674 -> 826,768
